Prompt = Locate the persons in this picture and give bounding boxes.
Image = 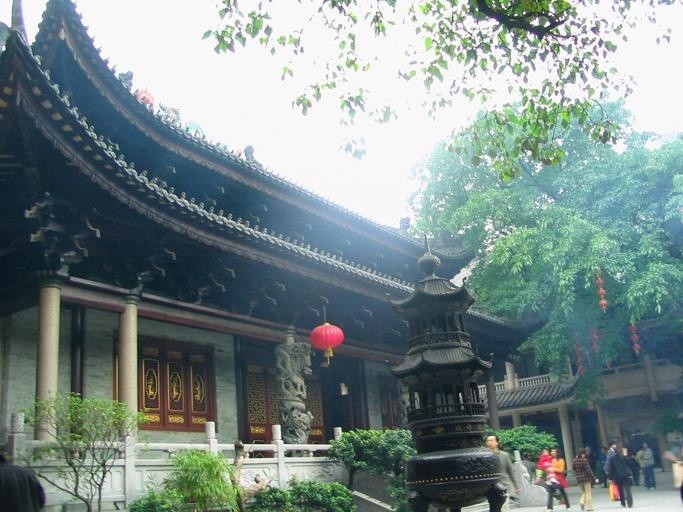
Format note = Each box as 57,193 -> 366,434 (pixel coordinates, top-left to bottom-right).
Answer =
546,448 -> 570,512
573,440 -> 683,512
0,456 -> 46,512
538,447 -> 560,486
486,434 -> 521,512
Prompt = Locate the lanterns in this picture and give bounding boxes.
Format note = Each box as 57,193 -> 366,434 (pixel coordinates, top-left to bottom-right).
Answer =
629,325 -> 641,358
310,323 -> 344,368
595,279 -> 609,314
576,328 -> 612,375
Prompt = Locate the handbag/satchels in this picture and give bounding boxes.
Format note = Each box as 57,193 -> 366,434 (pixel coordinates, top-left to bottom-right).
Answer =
609,482 -> 620,501
